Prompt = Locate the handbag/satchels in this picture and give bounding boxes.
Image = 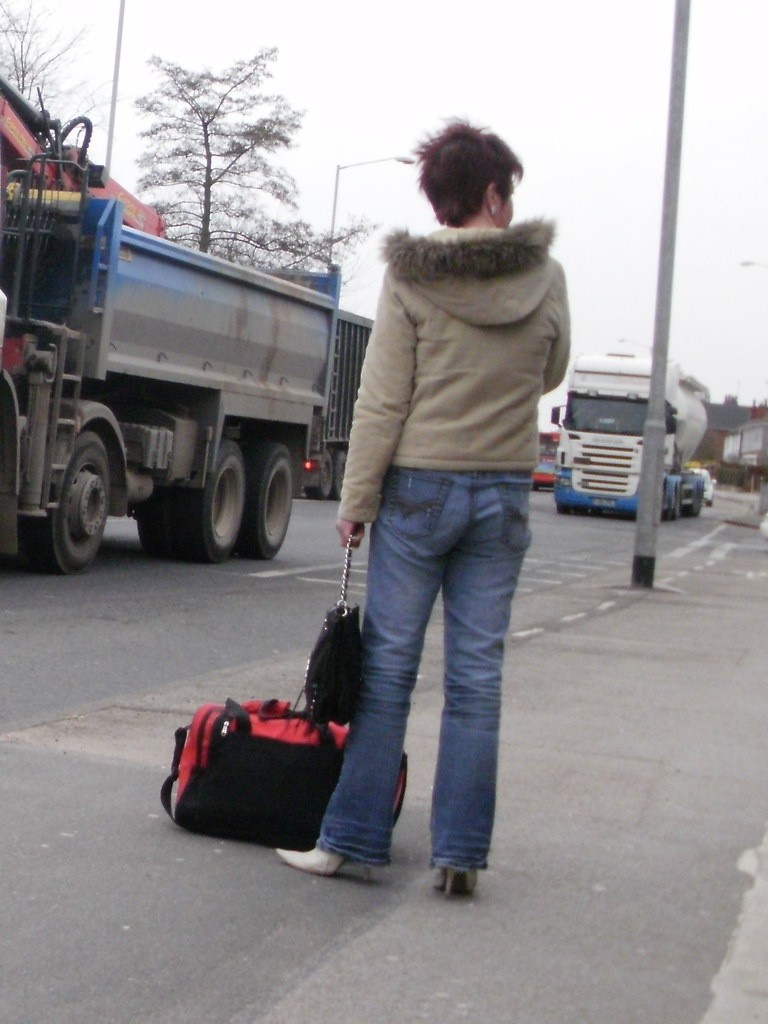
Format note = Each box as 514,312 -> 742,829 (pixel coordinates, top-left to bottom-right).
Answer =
159,697 -> 409,850
302,533 -> 361,725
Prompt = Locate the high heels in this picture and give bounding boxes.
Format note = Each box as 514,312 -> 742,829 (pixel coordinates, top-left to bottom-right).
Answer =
432,868 -> 475,897
273,844 -> 373,881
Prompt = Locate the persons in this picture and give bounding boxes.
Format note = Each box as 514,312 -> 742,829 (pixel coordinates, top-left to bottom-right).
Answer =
273,118 -> 574,899
